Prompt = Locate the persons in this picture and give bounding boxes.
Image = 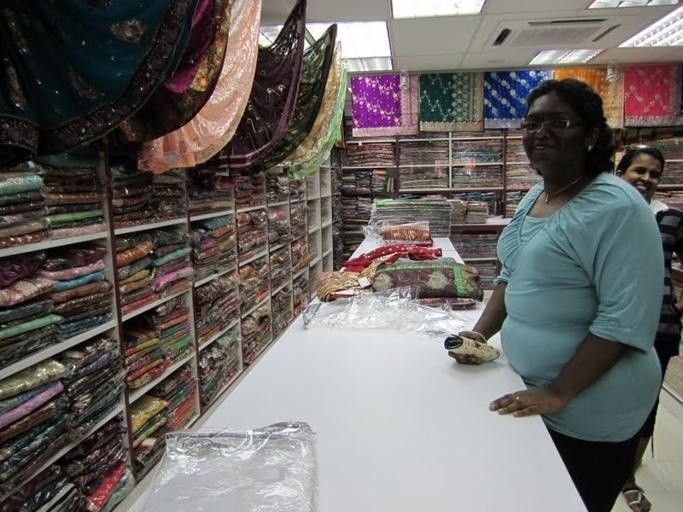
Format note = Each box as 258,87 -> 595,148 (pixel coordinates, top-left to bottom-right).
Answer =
447,76 -> 665,512
614,144 -> 682,511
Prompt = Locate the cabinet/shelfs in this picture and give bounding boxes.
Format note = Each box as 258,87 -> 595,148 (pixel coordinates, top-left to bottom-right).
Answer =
1,62 -> 339,511
319,60 -> 683,292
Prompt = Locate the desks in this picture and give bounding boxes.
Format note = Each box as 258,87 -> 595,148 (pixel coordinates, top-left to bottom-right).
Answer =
122,232 -> 590,511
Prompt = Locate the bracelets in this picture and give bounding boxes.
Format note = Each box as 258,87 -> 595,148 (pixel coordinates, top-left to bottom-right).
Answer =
472,330 -> 487,343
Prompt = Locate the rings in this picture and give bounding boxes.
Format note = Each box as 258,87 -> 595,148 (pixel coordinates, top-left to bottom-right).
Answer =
514,395 -> 523,407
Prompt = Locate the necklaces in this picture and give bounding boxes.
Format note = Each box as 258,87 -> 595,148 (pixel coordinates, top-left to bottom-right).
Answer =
538,173 -> 590,205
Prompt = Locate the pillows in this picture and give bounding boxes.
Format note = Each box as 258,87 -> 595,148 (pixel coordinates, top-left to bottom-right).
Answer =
371,261 -> 484,302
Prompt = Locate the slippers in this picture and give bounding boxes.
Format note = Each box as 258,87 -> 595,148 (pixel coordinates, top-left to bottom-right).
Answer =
624,486 -> 651,512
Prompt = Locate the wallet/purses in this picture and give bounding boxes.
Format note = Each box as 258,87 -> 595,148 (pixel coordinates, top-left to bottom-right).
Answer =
443,334 -> 501,363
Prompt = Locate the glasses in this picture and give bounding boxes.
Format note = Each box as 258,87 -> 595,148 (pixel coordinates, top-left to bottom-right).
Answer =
519,119 -> 584,130
